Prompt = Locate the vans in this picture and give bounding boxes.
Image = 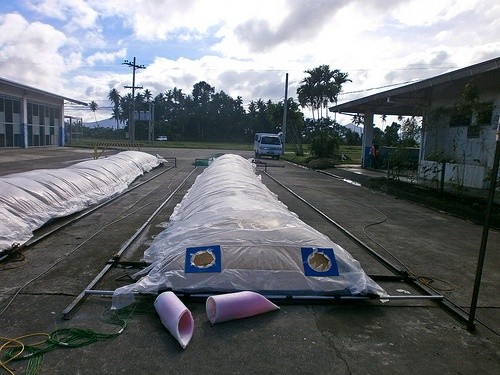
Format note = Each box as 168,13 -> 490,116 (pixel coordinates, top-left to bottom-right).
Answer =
157,135 -> 168,142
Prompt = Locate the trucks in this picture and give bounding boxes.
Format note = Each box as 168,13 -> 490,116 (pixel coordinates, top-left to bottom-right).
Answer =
253,132 -> 283,160
